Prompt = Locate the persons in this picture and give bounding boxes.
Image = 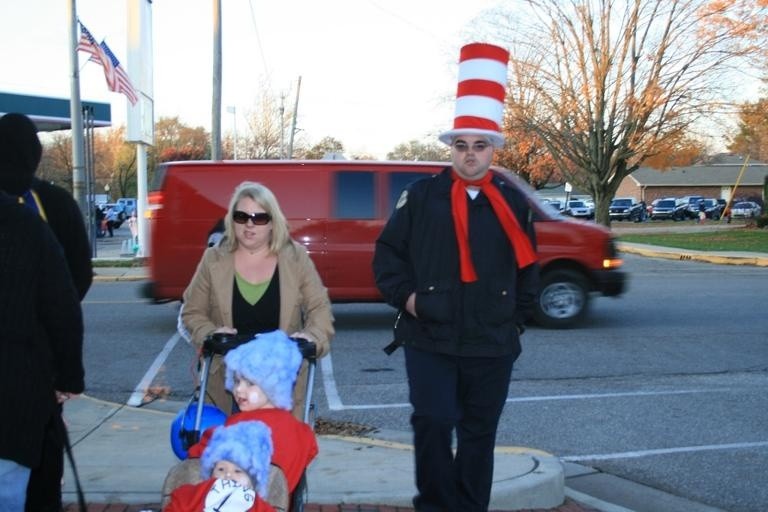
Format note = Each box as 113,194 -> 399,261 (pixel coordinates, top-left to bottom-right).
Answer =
20,177 -> 94,304
94,204 -> 130,239
159,418 -> 280,512
370,134 -> 541,512
1,110 -> 87,511
176,178 -> 337,420
696,199 -> 705,225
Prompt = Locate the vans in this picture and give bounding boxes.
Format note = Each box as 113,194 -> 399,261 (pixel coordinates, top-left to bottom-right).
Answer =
115,198 -> 135,217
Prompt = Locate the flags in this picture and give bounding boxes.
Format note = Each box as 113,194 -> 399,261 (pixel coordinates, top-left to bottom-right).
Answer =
80,24 -> 114,87
90,35 -> 141,108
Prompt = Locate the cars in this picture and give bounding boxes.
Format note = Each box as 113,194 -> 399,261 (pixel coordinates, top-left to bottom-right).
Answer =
545,195 -> 766,225
99,202 -> 127,225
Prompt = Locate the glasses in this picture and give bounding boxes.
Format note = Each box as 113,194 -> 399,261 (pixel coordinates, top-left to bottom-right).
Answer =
455,142 -> 491,153
232,210 -> 270,226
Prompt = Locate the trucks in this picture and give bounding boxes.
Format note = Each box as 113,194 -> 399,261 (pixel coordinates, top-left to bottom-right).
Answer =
144,158 -> 625,329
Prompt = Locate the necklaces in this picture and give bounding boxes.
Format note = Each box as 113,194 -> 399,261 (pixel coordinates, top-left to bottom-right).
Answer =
238,246 -> 271,257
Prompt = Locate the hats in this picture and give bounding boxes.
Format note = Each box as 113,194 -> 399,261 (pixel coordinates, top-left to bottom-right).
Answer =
439,43 -> 509,147
223,330 -> 303,411
198,419 -> 274,497
